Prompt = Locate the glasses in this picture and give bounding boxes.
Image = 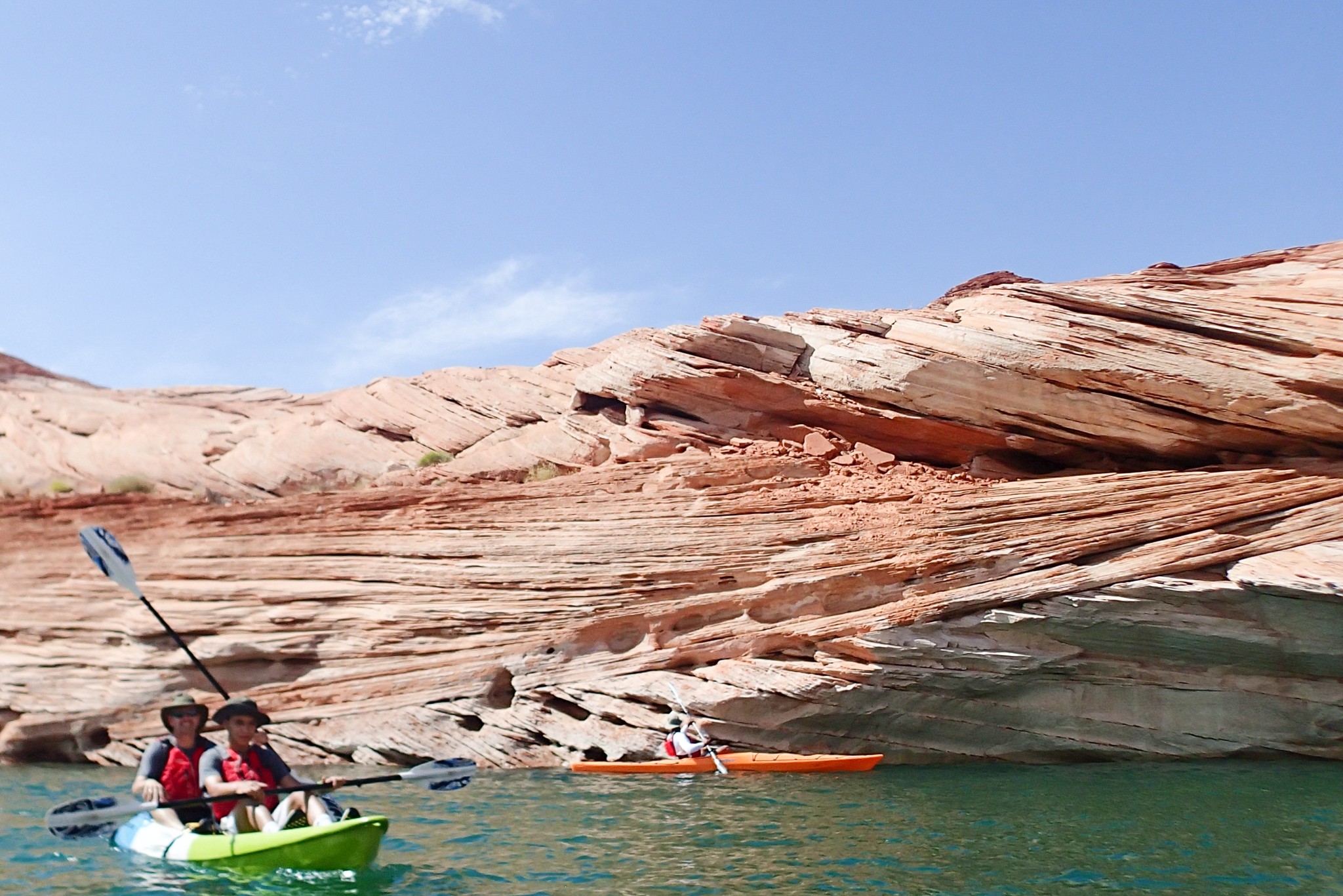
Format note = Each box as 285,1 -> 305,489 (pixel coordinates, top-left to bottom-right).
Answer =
166,709 -> 200,719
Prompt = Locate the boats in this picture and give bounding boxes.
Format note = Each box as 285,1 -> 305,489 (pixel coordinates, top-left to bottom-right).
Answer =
572,753 -> 884,773
109,810 -> 390,872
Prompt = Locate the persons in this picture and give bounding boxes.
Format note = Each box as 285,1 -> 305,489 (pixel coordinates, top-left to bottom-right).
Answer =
198,697 -> 361,833
132,695 -> 269,834
665,715 -> 734,760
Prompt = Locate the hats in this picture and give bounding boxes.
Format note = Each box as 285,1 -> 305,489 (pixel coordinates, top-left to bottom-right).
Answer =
211,696 -> 271,725
663,710 -> 687,730
161,693 -> 208,734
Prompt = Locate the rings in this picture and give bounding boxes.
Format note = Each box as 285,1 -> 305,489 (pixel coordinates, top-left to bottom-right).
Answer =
148,789 -> 153,792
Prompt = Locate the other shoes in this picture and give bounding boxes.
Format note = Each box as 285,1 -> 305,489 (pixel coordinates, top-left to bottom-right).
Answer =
279,806 -> 310,830
341,807 -> 360,822
185,818 -> 214,835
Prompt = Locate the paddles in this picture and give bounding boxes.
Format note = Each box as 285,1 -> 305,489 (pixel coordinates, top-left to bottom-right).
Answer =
47,756 -> 478,843
78,524 -> 346,824
664,681 -> 728,772
